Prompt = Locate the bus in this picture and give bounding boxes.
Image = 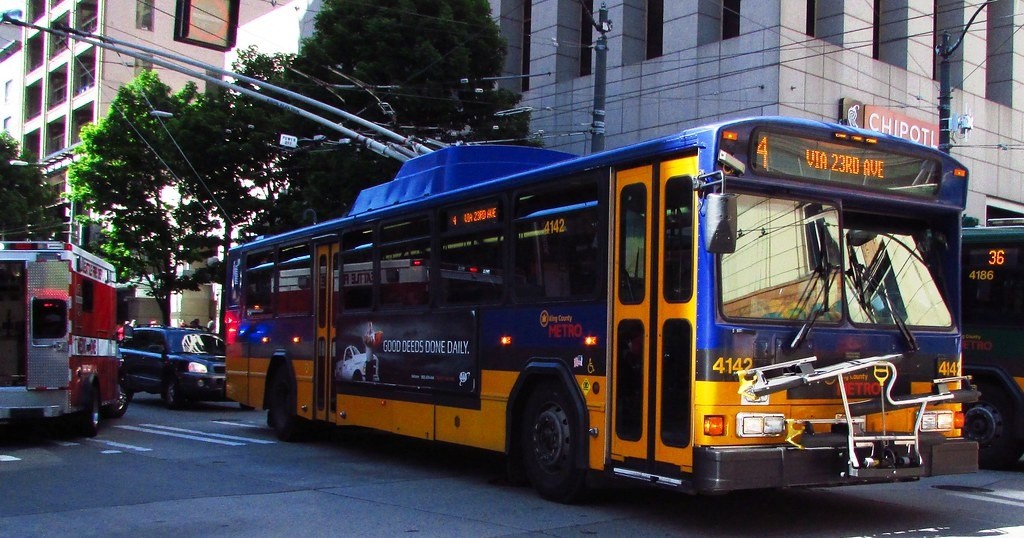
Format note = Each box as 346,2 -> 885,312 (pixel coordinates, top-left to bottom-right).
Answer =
221,113 -> 972,511
948,210 -> 1024,476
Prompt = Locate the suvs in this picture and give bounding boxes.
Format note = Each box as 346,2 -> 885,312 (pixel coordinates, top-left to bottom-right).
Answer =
121,327 -> 255,412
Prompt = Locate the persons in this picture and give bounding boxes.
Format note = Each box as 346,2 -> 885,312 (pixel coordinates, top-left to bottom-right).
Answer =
191,318 -> 204,330
120,318 -> 161,329
204,315 -> 216,334
363,320 -> 376,382
187,321 -> 199,330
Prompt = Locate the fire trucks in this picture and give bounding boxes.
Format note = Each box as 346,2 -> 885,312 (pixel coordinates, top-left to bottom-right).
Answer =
1,240 -> 133,439
